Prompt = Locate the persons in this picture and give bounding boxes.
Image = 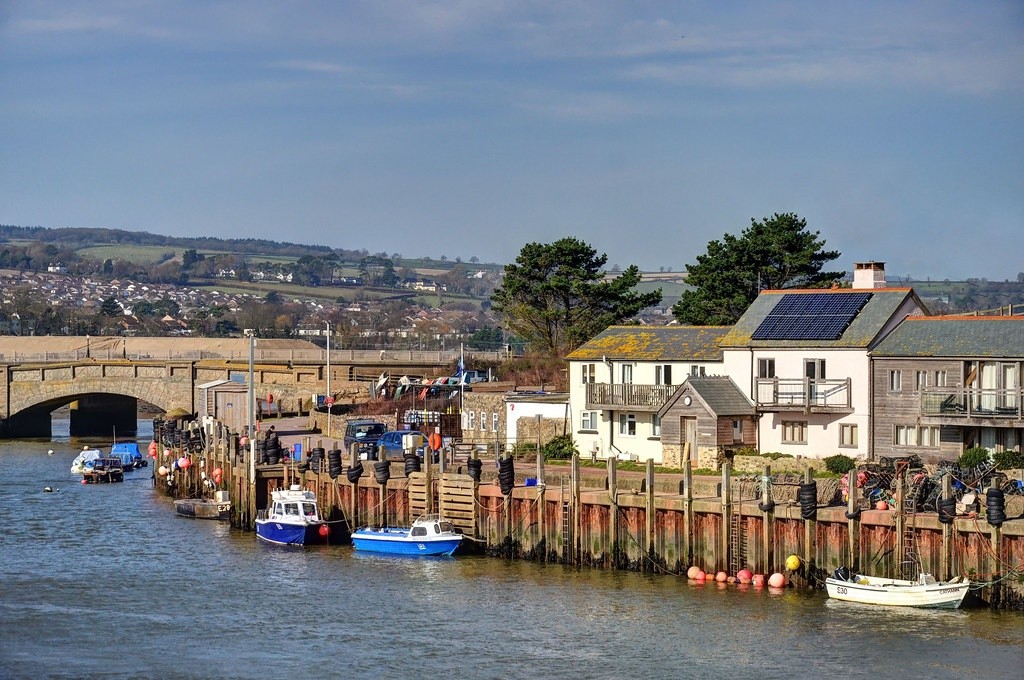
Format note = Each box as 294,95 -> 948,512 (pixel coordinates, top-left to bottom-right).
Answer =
266,425 -> 275,439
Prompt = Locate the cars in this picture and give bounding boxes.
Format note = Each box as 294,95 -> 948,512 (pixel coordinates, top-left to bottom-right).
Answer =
437,370 -> 498,384
377,430 -> 440,464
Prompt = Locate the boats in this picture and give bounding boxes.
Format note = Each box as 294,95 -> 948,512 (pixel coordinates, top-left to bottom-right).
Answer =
70,450 -> 104,476
108,443 -> 142,472
254,451 -> 325,548
173,498 -> 232,520
82,458 -> 123,483
350,512 -> 464,557
826,565 -> 972,609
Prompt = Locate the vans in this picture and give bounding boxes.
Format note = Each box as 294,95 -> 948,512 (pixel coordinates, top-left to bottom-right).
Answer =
344,418 -> 388,460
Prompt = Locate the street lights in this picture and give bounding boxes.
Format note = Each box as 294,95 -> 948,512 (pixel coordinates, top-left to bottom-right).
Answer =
322,320 -> 330,438
